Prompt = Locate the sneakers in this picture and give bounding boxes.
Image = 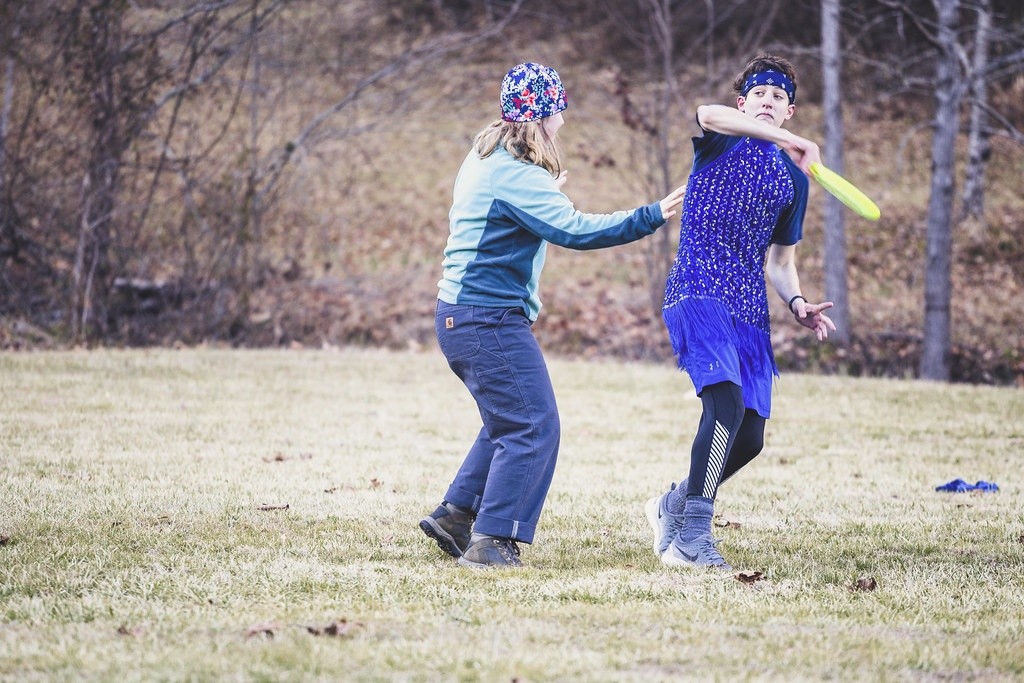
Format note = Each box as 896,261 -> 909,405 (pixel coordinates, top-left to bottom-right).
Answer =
644,482 -> 685,558
661,532 -> 734,572
418,499 -> 476,560
457,531 -> 523,572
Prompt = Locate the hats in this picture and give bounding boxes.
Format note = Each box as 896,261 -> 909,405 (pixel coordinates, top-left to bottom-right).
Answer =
499,63 -> 568,122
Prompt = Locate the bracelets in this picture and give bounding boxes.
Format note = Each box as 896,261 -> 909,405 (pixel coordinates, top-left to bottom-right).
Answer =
789,296 -> 808,313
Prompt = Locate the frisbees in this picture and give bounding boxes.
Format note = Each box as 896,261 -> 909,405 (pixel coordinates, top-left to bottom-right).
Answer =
809,162 -> 881,220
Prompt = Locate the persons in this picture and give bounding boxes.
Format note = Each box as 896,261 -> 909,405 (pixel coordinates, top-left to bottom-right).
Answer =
419,62 -> 687,568
644,54 -> 837,575
